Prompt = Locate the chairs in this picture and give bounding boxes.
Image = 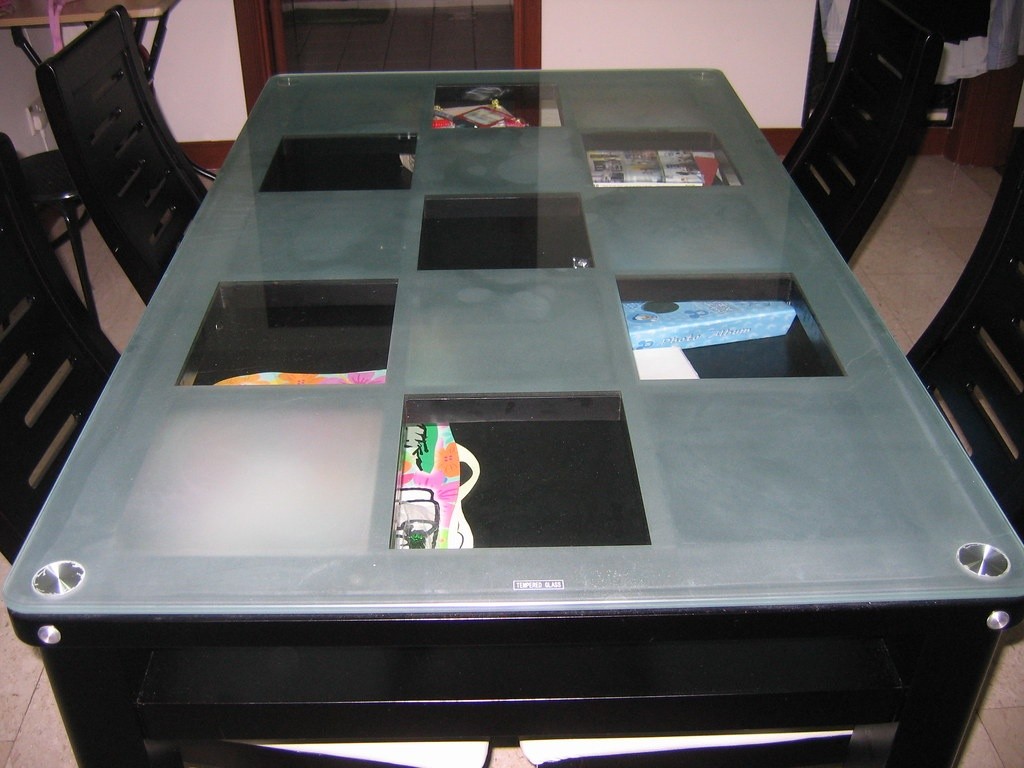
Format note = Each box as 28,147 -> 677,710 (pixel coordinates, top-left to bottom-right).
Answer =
780,0 -> 945,268
34,4 -> 216,306
0,130 -> 123,564
906,123 -> 1024,647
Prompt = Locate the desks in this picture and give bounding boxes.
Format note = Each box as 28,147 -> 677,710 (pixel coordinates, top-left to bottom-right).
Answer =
0,0 -> 217,242
9,68 -> 1022,768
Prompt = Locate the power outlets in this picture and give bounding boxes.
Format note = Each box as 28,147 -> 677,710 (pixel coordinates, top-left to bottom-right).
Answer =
26,96 -> 47,137
